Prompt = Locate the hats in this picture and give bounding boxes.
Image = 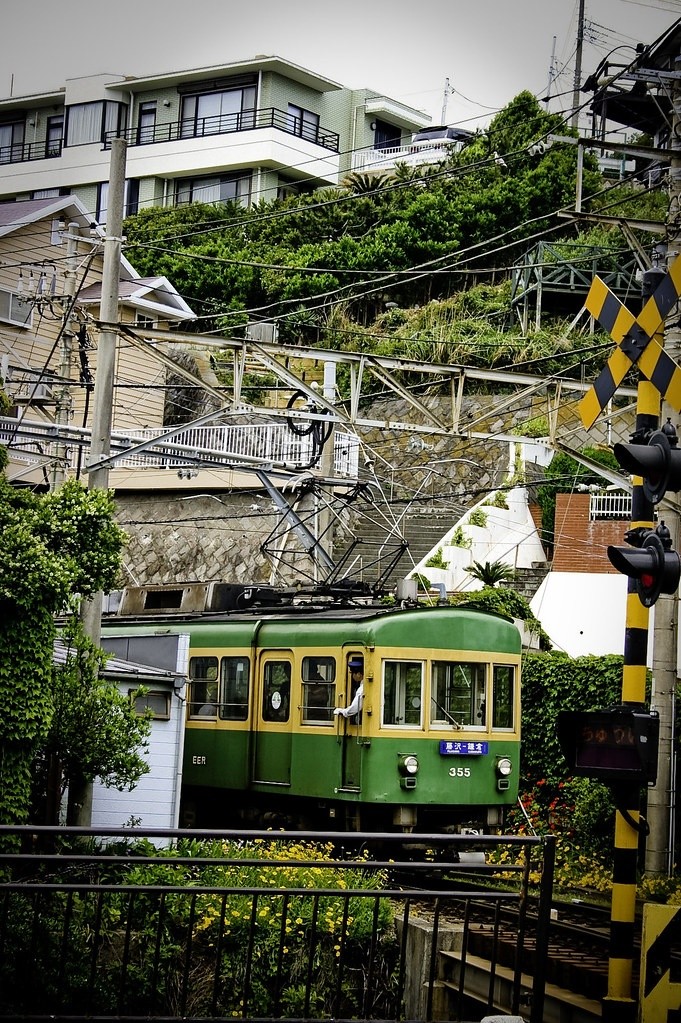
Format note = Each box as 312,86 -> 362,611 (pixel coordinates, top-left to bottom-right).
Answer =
347,661 -> 363,674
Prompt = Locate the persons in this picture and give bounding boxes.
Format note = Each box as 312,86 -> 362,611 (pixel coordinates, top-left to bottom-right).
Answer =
333,661 -> 364,725
279,659 -> 329,719
198,685 -> 217,716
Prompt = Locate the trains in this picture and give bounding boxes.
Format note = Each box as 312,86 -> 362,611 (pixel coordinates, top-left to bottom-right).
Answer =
48,470 -> 523,851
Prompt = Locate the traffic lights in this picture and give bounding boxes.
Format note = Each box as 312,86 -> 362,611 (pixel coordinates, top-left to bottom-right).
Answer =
607,525 -> 681,607
613,429 -> 681,493
556,703 -> 657,786
